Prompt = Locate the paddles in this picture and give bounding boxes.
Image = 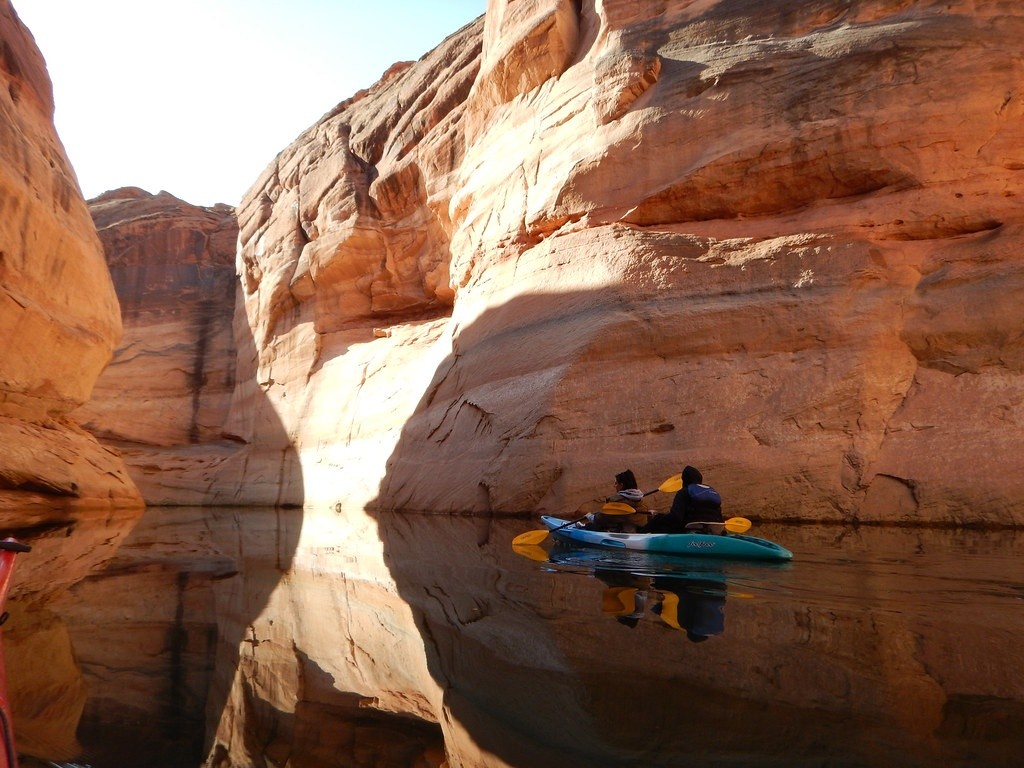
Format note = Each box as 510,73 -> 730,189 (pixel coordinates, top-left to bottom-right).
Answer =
511,472 -> 682,545
600,502 -> 752,533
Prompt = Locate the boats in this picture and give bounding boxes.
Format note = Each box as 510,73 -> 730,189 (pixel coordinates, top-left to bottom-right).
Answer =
540,515 -> 794,559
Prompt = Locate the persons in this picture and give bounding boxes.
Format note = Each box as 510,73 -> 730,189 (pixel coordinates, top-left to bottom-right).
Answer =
576,469 -> 648,533
635,465 -> 727,537
